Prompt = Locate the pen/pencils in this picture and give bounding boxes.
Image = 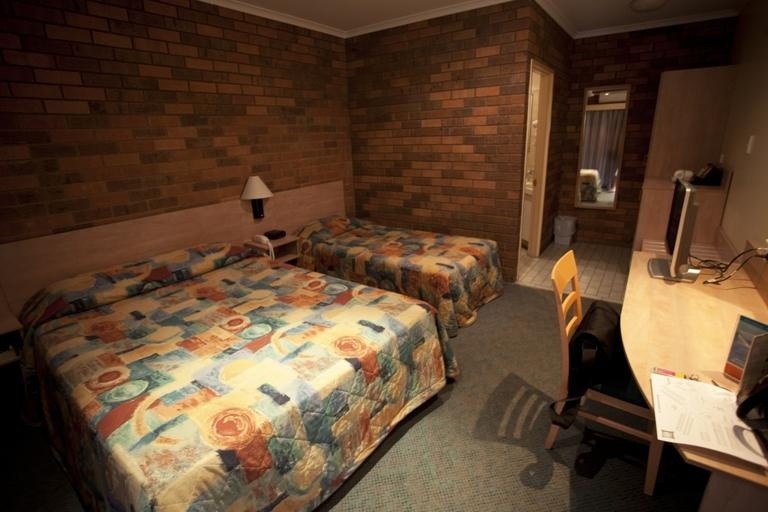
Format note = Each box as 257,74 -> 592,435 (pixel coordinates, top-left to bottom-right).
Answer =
654,366 -> 698,381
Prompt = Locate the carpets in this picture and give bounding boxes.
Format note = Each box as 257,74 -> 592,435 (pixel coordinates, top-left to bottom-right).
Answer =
318,279 -> 703,512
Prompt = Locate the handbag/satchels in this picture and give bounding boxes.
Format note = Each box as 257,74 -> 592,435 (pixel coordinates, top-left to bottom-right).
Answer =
568,300 -> 626,396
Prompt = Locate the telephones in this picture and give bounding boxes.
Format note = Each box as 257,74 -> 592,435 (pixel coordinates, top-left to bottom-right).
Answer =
254,235 -> 269,245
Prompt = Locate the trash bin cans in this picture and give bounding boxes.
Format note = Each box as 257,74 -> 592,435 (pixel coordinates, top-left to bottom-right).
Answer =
553,215 -> 578,246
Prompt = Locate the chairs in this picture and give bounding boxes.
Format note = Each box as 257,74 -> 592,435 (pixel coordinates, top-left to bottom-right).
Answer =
545,249 -> 666,494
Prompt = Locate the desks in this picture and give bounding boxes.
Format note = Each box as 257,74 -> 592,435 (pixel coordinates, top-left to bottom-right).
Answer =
619,251 -> 768,489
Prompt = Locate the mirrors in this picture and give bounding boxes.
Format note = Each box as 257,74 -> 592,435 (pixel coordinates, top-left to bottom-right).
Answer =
574,83 -> 631,212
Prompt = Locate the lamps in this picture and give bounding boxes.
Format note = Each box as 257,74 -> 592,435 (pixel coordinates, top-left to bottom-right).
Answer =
241,176 -> 274,219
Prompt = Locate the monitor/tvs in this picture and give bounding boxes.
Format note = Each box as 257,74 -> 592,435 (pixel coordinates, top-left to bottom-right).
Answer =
647,176 -> 702,283
